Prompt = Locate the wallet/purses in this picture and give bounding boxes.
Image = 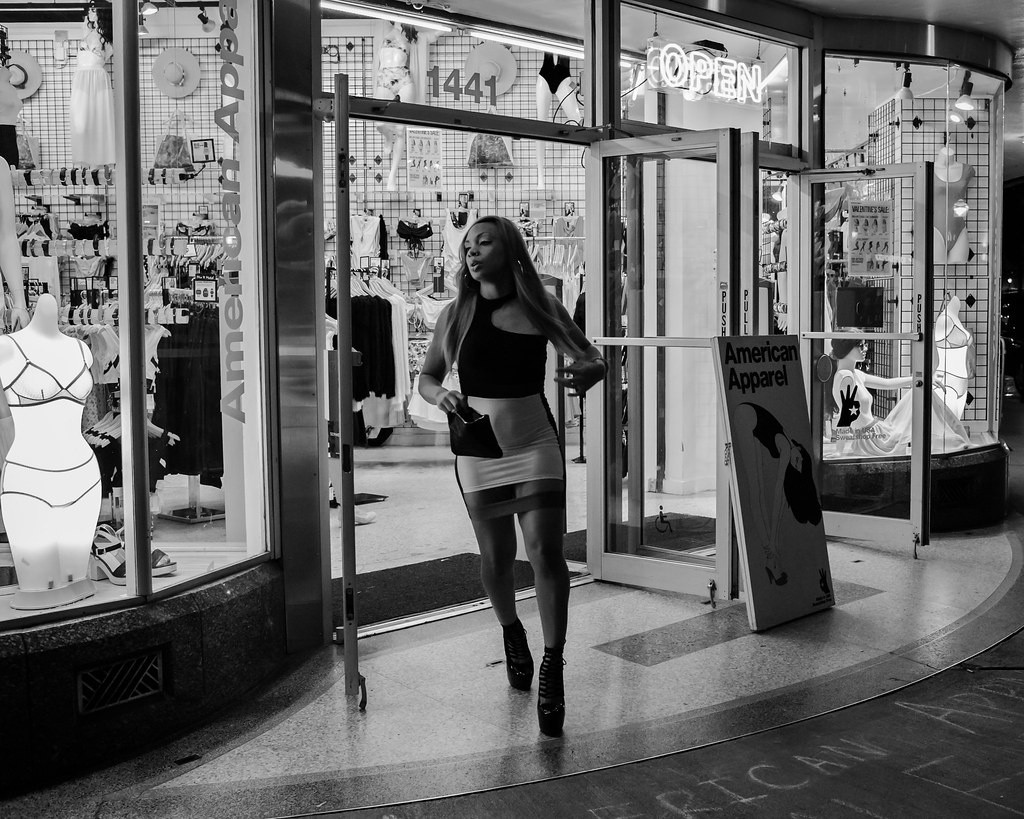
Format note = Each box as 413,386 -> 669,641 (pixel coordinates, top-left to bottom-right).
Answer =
446,406 -> 504,459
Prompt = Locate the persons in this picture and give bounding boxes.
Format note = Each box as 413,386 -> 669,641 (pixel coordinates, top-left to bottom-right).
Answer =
417,214 -> 611,736
0,28 -> 40,479
0,286 -> 102,596
366,10 -> 445,192
65,0 -> 133,172
830,327 -> 976,459
930,297 -> 976,441
532,39 -> 588,191
928,148 -> 976,265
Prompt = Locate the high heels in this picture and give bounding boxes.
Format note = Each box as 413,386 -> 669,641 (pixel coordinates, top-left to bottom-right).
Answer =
539,642 -> 565,736
501,619 -> 534,691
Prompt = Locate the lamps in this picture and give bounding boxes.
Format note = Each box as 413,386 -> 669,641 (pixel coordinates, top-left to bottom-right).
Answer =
951,80 -> 977,127
138,0 -> 160,39
894,72 -> 914,102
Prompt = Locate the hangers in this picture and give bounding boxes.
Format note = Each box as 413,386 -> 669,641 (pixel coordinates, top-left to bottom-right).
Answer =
326,264 -> 405,300
146,236 -> 230,296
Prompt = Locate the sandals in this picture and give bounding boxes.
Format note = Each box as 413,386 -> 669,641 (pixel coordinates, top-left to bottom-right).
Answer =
90,526 -> 126,586
97,523 -> 178,576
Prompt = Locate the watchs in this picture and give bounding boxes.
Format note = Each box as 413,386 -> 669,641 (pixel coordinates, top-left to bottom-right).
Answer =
590,357 -> 610,377
